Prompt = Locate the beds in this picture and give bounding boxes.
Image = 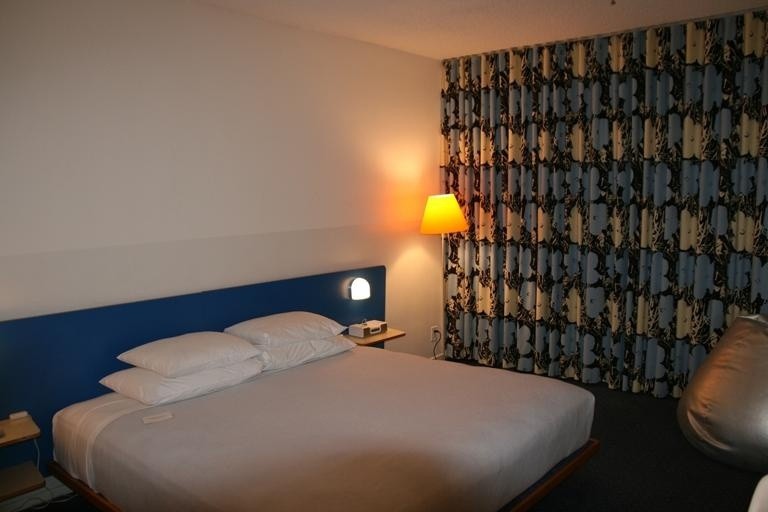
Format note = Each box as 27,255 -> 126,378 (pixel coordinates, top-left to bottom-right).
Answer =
51,346 -> 601,512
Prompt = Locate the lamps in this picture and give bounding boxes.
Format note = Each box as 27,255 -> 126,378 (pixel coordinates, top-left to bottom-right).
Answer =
420,193 -> 469,360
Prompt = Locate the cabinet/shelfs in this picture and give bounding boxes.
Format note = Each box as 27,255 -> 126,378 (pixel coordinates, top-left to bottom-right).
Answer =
0,415 -> 45,503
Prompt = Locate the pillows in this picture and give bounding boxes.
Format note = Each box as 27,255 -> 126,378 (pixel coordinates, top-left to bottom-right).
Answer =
98,311 -> 357,409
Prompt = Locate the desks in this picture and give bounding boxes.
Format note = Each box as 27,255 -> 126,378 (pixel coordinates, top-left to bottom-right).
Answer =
343,327 -> 405,346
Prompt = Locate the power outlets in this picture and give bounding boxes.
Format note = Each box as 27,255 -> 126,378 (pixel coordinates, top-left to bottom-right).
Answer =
430,325 -> 440,341
